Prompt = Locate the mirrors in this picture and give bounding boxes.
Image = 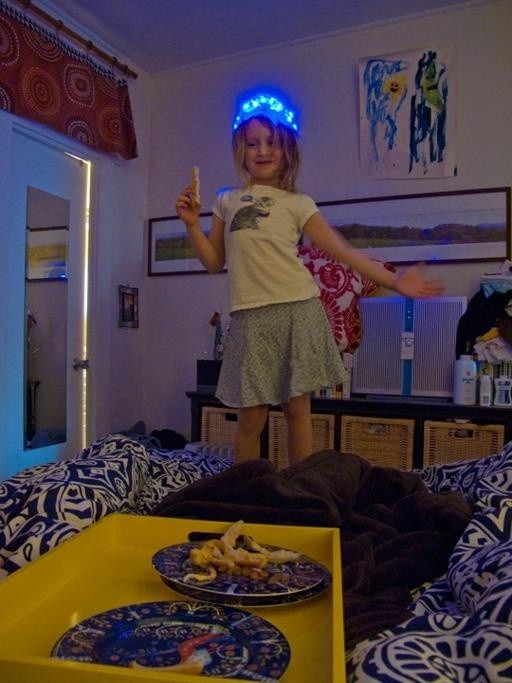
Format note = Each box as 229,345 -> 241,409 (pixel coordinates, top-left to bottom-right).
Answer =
22,185 -> 70,453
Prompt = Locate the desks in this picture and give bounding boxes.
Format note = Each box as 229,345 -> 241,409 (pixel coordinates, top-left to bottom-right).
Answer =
185,385 -> 511,470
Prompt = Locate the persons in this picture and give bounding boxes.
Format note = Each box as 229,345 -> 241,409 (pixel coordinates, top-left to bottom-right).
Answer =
175,86 -> 446,471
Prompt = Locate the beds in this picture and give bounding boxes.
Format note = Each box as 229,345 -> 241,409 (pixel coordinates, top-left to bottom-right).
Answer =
2,432 -> 512,683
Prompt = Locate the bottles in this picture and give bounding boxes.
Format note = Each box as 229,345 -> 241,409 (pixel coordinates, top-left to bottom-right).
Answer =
451,353 -> 512,406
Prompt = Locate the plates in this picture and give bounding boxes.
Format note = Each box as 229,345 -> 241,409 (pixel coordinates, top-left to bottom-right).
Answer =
159,564 -> 333,608
49,597 -> 291,683
149,538 -> 327,597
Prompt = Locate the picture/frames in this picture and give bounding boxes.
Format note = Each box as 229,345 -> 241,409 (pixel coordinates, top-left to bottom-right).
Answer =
146,185 -> 511,279
26,225 -> 68,282
